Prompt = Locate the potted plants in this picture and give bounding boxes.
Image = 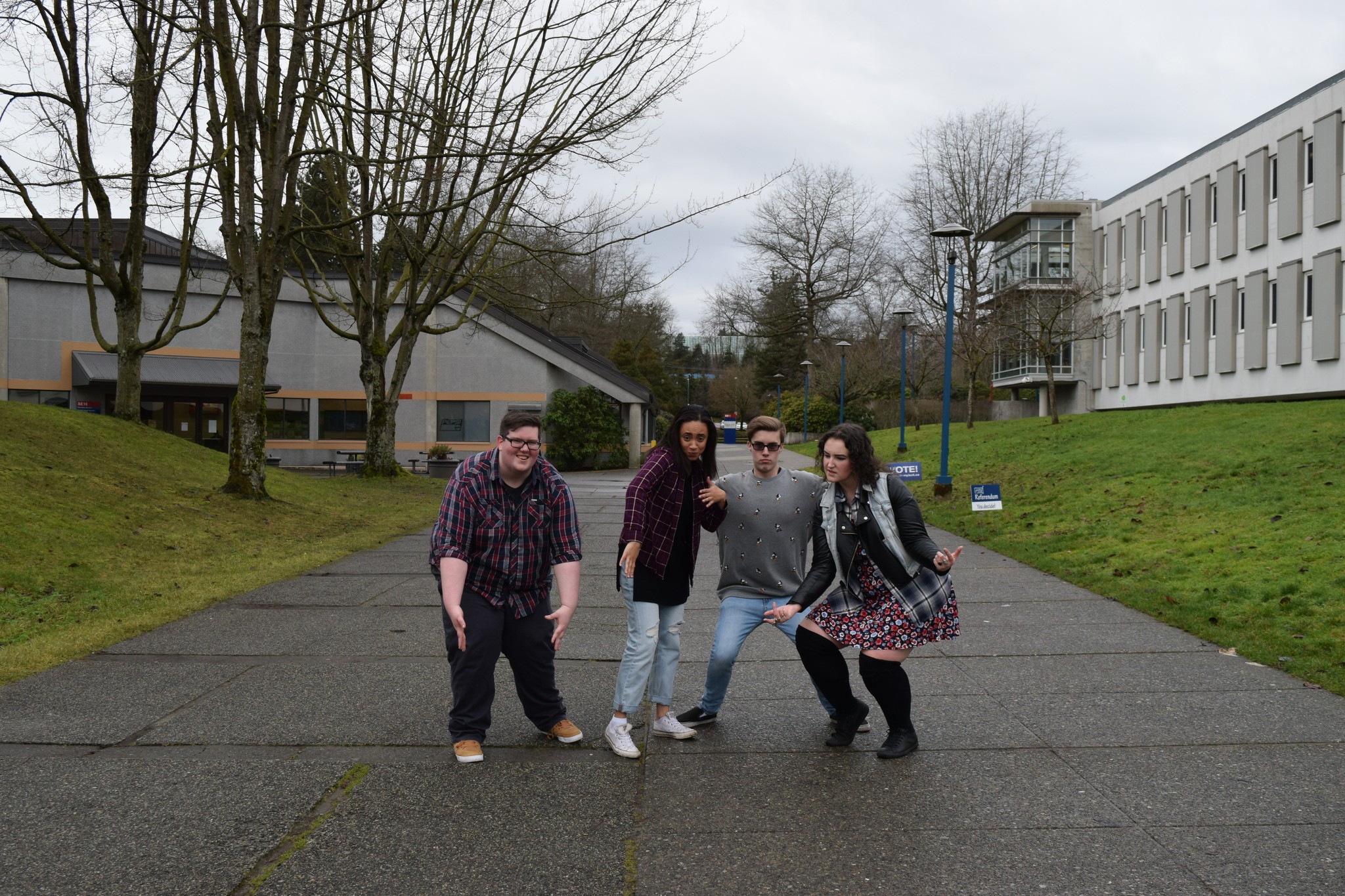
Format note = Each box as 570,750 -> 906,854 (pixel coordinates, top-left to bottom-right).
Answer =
427,444 -> 460,480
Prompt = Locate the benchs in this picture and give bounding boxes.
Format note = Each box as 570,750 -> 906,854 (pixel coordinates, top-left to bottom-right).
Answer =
323,461 -> 364,476
408,459 -> 420,473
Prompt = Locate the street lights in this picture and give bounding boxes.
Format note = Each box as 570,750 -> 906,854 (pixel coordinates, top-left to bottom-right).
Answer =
892,305 -> 916,455
773,374 -> 785,420
835,340 -> 852,424
929,223 -> 975,500
735,377 -> 738,413
688,373 -> 691,405
800,360 -> 814,444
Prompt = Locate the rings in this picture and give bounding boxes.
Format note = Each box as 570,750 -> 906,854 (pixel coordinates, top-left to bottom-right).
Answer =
781,614 -> 784,617
938,560 -> 943,565
771,619 -> 777,626
942,559 -> 947,564
775,617 -> 780,622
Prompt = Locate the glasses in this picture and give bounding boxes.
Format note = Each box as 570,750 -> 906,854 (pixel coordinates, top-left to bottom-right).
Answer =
750,440 -> 781,451
505,436 -> 542,450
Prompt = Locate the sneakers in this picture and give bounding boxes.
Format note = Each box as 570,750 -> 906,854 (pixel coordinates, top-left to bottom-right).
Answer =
877,728 -> 919,758
542,719 -> 583,743
653,711 -> 698,739
453,739 -> 484,763
826,699 -> 869,747
605,721 -> 641,758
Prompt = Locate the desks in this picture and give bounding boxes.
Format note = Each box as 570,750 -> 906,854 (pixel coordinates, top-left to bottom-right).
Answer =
420,451 -> 455,473
337,451 -> 365,474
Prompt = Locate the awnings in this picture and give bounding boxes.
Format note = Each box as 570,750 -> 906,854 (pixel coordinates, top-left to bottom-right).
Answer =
71,350 -> 282,394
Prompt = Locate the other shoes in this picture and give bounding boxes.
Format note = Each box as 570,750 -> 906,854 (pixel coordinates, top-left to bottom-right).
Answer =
676,707 -> 719,728
828,714 -> 870,732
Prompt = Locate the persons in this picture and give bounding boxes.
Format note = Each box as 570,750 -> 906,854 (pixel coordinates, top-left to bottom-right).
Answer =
429,410 -> 583,762
764,425 -> 963,759
675,416 -> 871,732
604,406 -> 727,758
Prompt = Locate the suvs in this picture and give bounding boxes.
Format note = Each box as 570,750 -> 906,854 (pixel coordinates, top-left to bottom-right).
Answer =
720,420 -> 724,430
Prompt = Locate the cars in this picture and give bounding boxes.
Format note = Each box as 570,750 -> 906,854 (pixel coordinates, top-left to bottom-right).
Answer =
736,422 -> 748,431
714,423 -> 721,430
